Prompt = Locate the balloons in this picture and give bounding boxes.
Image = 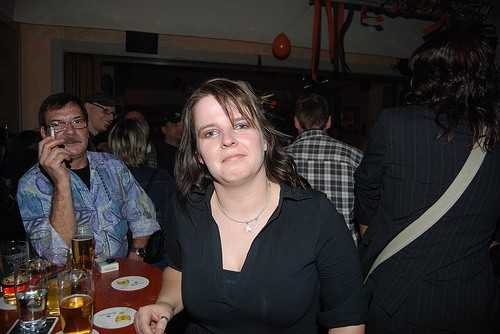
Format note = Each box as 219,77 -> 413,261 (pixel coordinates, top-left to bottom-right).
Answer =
272,33 -> 291,60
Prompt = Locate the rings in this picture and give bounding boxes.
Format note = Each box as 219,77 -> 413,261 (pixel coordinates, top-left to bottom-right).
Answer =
161,316 -> 168,320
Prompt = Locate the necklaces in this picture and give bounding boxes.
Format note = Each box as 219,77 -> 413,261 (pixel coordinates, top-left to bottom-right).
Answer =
217,182 -> 272,232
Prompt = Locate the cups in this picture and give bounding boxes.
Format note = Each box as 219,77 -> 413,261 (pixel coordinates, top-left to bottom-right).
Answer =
71,228 -> 95,272
0,241 -> 29,305
14,259 -> 48,331
42,247 -> 73,315
57,269 -> 94,334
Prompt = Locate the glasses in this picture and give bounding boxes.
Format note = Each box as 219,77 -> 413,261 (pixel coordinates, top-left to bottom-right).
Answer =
43,119 -> 88,132
89,103 -> 117,119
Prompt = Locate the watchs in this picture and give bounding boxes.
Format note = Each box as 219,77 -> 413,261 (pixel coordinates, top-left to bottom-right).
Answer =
131,248 -> 146,257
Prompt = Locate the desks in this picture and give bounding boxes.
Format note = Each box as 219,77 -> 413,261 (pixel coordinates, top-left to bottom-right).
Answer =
0,258 -> 163,334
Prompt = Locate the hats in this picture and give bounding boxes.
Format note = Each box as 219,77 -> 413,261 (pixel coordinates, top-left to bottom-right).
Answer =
162,113 -> 182,124
83,93 -> 122,113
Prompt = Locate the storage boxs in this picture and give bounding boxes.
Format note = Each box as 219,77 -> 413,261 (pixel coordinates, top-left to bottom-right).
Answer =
94,258 -> 119,273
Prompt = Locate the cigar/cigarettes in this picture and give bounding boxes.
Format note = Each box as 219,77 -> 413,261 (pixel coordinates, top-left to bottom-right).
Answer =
50,127 -> 55,140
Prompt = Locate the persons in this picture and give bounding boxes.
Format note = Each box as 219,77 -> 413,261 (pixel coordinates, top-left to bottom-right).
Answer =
16,93 -> 162,266
281,93 -> 367,248
84,90 -> 185,189
134,78 -> 367,334
352,11 -> 500,334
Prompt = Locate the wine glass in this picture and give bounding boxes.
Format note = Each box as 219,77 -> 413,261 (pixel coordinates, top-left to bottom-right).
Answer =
28,230 -> 53,290
